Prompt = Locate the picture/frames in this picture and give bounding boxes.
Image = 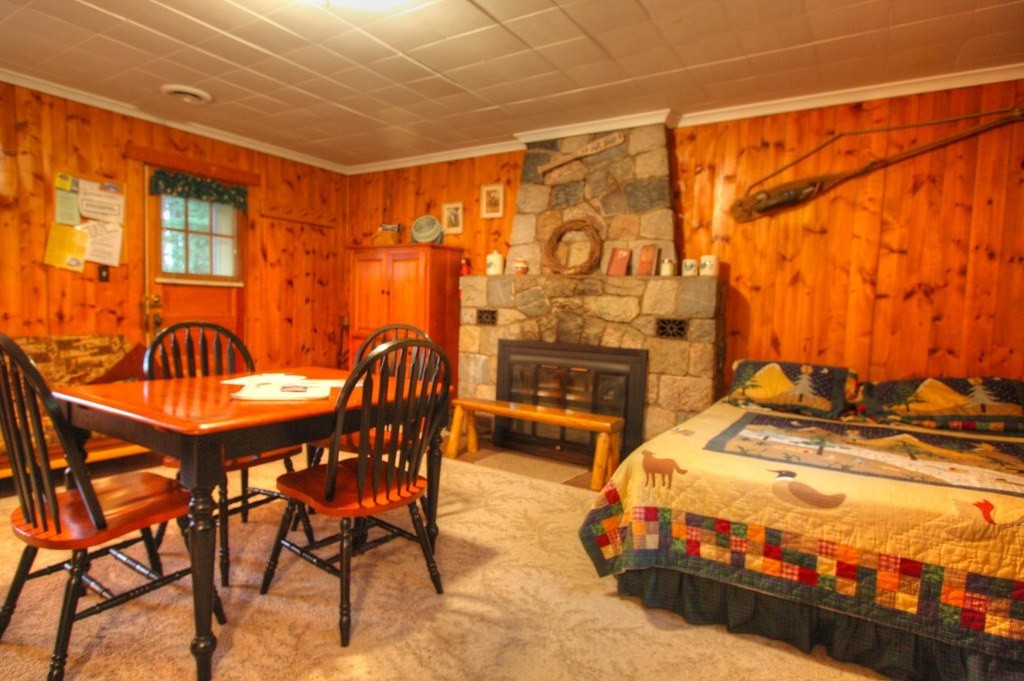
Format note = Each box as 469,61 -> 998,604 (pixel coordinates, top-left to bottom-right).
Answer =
479,184 -> 503,218
441,201 -> 463,233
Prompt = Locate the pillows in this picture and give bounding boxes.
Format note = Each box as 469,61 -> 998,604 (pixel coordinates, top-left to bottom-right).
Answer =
728,359 -> 858,419
859,377 -> 1024,432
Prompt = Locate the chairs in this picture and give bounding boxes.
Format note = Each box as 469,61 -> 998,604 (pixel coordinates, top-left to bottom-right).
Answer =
260,339 -> 451,646
0,331 -> 227,681
292,323 -> 434,531
142,322 -> 314,586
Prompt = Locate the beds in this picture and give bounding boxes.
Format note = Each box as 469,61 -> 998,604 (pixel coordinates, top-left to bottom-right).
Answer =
579,395 -> 1024,681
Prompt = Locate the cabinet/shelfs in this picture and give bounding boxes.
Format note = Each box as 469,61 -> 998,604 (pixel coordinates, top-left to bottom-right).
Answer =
345,244 -> 464,406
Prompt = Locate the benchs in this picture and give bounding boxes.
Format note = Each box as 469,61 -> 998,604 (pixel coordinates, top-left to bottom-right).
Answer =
446,397 -> 625,491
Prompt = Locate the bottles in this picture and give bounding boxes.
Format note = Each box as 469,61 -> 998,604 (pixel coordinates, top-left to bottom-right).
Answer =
659,258 -> 673,276
456,257 -> 471,301
485,249 -> 504,276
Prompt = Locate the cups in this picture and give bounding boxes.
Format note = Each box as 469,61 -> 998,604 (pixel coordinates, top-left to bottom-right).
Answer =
682,258 -> 698,277
699,254 -> 719,276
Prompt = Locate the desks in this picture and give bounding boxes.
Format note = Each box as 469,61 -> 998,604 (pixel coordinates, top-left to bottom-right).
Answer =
49,366 -> 454,681
